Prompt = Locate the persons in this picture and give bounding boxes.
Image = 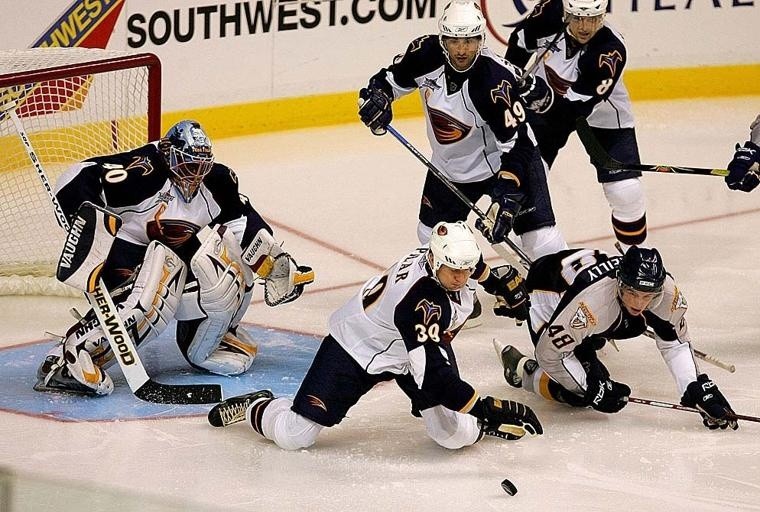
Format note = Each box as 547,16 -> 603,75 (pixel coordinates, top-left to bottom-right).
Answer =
501,245 -> 739,430
504,2 -> 648,250
725,121 -> 756,191
358,2 -> 568,316
208,221 -> 543,452
38,120 -> 314,397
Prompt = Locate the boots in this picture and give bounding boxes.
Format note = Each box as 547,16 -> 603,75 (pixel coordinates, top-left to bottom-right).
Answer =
501,345 -> 538,389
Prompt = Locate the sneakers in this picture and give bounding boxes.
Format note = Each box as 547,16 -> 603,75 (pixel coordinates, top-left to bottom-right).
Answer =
208,389 -> 274,428
37,354 -> 94,393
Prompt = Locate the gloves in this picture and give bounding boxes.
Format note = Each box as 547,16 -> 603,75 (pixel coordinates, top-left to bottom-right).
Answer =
475,193 -> 527,244
477,395 -> 543,441
582,372 -> 631,414
358,88 -> 392,136
680,373 -> 739,430
490,265 -> 531,326
725,141 -> 760,192
517,73 -> 554,114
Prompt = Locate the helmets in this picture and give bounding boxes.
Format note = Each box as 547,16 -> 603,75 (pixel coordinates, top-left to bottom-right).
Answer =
617,246 -> 665,311
438,1 -> 487,72
562,0 -> 609,32
159,120 -> 215,203
425,221 -> 480,292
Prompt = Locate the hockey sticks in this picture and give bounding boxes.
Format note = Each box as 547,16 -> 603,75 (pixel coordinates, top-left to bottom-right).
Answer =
517,11 -> 574,86
492,243 -> 735,376
571,115 -> 732,177
0,101 -> 223,402
